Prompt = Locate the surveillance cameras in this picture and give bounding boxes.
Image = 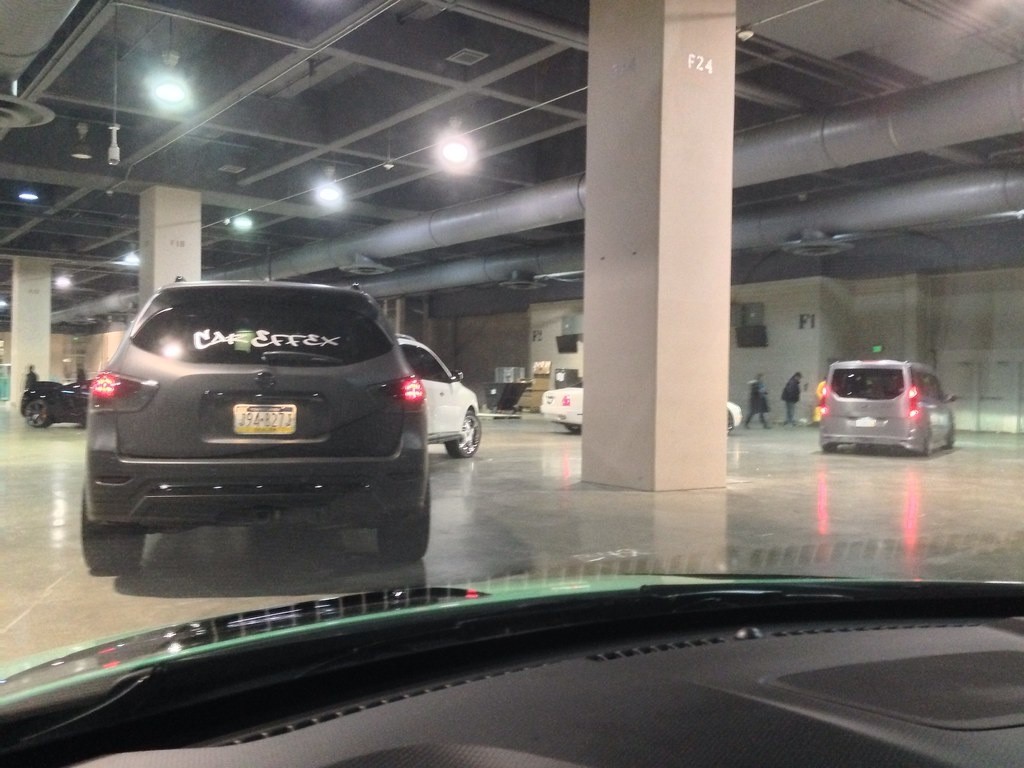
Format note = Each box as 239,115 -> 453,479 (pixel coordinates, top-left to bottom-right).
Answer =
107,146 -> 121,166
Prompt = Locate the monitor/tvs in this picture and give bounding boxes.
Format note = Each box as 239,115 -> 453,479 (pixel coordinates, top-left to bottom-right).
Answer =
735,325 -> 768,348
556,334 -> 576,353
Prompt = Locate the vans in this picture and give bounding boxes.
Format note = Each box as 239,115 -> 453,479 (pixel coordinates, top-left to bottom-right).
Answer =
820,358 -> 956,456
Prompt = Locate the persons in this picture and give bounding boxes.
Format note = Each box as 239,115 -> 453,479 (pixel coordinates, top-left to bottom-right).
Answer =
781,372 -> 802,425
743,374 -> 770,428
25,366 -> 36,389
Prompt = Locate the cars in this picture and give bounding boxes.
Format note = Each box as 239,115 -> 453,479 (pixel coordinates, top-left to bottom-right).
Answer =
17,383 -> 85,428
541,378 -> 741,433
395,331 -> 482,459
81,280 -> 428,563
